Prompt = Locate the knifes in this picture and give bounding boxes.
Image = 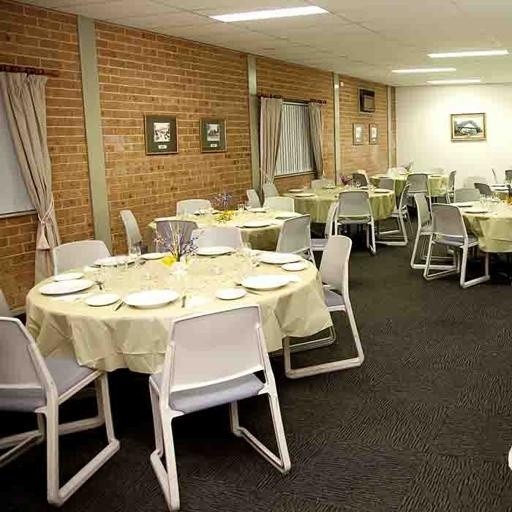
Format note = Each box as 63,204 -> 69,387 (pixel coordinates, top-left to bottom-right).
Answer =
182,296 -> 186,308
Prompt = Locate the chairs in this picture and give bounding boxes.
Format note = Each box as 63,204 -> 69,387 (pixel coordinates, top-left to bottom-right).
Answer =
144,299 -> 294,512
423,202 -> 490,289
0,284 -> 15,320
270,234 -> 367,388
46,239 -> 110,276
106,165 -> 457,256
1,316 -> 125,507
410,193 -> 458,270
455,167 -> 511,201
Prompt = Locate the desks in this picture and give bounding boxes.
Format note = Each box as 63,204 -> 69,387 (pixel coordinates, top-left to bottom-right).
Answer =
26,250 -> 339,383
448,201 -> 511,279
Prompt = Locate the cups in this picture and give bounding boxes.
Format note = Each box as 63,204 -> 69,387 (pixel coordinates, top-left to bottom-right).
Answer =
479,193 -> 507,215
321,175 -> 361,195
93,241 -> 263,292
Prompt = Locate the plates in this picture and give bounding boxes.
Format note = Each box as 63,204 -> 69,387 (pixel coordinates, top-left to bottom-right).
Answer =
282,263 -> 307,271
288,186 -> 390,197
214,288 -> 246,300
83,292 -> 121,306
449,202 -> 487,213
192,207 -> 297,228
55,272 -> 84,281
124,290 -> 180,308
239,277 -> 290,290
39,280 -> 94,295
262,253 -> 300,264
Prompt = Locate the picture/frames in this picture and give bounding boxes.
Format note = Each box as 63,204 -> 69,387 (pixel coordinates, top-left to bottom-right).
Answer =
142,112 -> 179,156
197,113 -> 228,154
368,122 -> 380,148
448,111 -> 488,144
352,121 -> 365,146
